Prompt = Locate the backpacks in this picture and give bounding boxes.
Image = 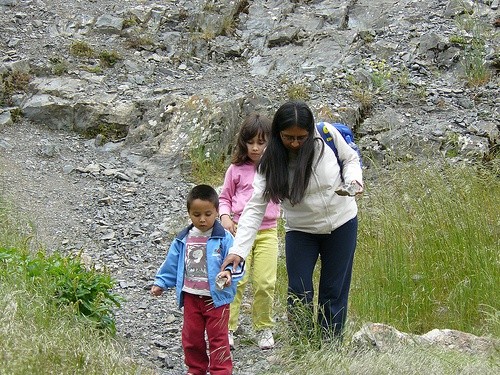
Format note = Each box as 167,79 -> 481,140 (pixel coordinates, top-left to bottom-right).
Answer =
316,121 -> 362,182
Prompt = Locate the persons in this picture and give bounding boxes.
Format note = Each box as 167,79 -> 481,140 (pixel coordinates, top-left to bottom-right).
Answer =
152,184 -> 245,375
218,114 -> 280,350
220,101 -> 365,350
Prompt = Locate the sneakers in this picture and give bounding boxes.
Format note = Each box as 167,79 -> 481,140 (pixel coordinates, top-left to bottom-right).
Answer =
228,330 -> 235,350
258,328 -> 274,349
